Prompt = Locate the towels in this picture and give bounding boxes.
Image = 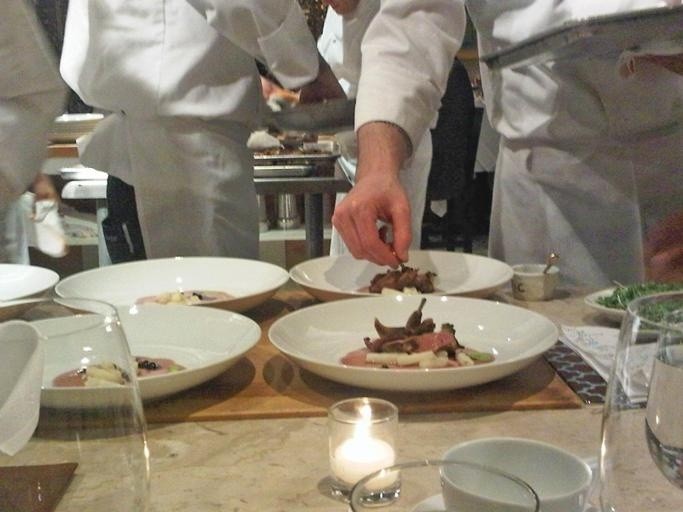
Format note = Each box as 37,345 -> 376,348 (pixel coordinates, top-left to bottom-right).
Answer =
557,325 -> 655,405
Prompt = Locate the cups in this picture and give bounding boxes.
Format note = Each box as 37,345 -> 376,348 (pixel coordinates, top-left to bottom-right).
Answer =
508,262 -> 561,300
324,397 -> 402,503
437,436 -> 593,510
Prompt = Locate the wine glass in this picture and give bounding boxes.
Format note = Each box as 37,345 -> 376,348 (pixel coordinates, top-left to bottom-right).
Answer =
595,292 -> 683,511
0,295 -> 154,512
344,458 -> 540,511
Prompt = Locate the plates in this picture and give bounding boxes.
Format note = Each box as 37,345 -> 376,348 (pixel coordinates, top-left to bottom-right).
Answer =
55,255 -> 288,314
290,249 -> 516,302
18,304 -> 262,409
0,257 -> 60,312
584,286 -> 638,320
267,292 -> 561,393
409,487 -> 601,512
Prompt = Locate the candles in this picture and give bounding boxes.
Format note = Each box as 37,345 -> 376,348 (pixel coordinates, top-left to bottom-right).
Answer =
331,402 -> 396,490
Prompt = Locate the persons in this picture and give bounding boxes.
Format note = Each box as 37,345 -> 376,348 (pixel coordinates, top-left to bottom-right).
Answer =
331,0 -> 683,306
57,0 -> 347,267
0,0 -> 71,268
318,0 -> 475,258
